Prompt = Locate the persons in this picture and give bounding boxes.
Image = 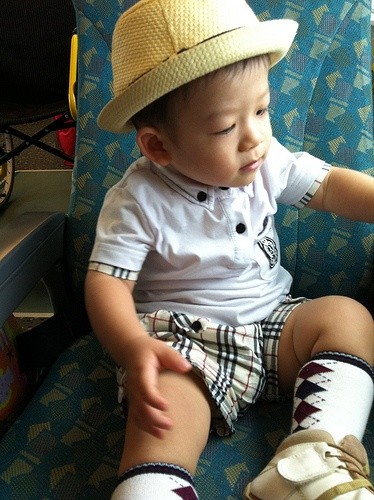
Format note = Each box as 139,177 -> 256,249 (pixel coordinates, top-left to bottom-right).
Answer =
85,0 -> 374,500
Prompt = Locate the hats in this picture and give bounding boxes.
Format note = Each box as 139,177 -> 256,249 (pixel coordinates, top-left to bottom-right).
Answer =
97,0 -> 299,133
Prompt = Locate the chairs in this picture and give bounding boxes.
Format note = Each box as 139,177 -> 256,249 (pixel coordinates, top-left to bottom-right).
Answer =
0,0 -> 374,500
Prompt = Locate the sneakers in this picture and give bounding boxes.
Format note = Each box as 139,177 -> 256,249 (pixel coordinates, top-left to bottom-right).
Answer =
244,429 -> 374,500
